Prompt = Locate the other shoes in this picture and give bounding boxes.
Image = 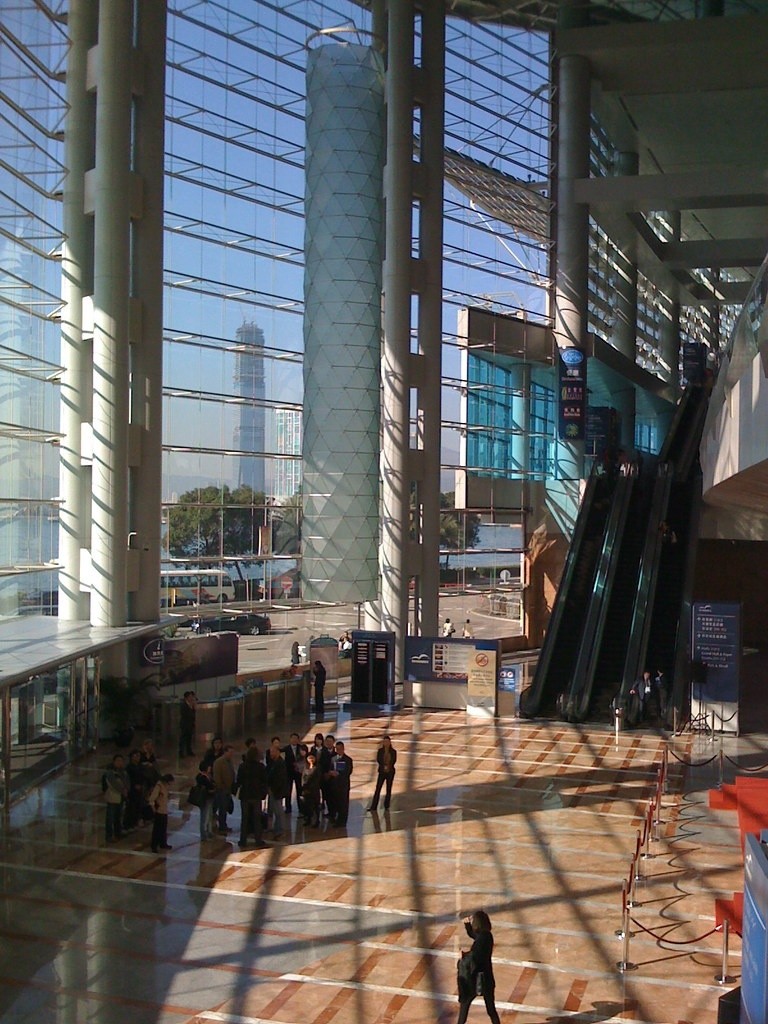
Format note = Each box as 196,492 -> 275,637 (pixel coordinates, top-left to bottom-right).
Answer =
238,841 -> 245,847
285,809 -> 291,813
297,803 -> 346,828
385,804 -> 390,808
152,846 -> 159,853
201,826 -> 232,844
106,817 -> 144,843
367,807 -> 375,811
256,841 -> 271,847
161,843 -> 172,849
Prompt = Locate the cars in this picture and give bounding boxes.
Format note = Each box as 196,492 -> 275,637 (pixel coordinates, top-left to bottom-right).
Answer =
191,611 -> 272,636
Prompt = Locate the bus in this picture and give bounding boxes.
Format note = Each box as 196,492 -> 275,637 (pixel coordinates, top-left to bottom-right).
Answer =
160,570 -> 236,609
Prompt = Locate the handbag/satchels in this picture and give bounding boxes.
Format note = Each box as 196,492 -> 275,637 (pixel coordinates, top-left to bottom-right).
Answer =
144,784 -> 161,811
187,786 -> 207,808
262,812 -> 268,830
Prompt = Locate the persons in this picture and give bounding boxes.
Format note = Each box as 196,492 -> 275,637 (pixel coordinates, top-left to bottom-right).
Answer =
313,660 -> 326,713
104,739 -> 174,853
178,696 -> 197,759
462,619 -> 473,639
291,641 -> 302,667
338,631 -> 353,659
306,635 -> 315,662
367,736 -> 396,811
457,911 -> 501,1024
196,733 -> 353,847
658,519 -> 677,562
630,667 -> 669,722
443,618 -> 454,638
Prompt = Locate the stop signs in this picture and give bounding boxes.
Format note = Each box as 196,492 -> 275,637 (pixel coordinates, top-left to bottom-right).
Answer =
281,576 -> 294,590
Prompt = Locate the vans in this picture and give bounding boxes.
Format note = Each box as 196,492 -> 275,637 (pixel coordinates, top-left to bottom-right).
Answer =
6,604 -> 59,618
